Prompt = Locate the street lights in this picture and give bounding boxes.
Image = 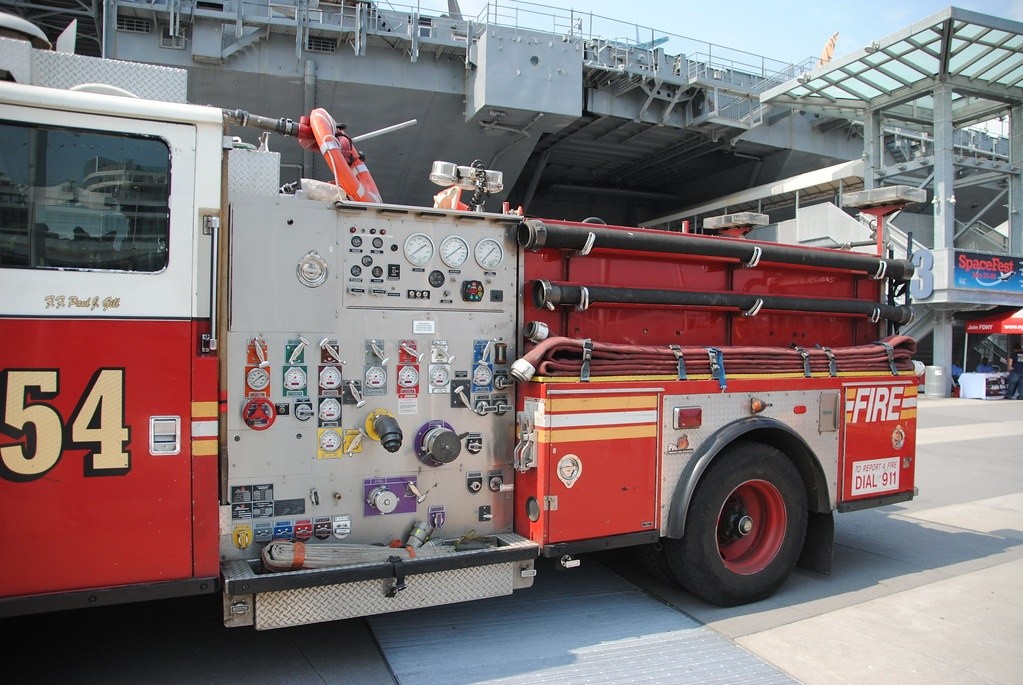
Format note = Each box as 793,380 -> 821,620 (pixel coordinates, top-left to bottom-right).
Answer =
133,185 -> 139,250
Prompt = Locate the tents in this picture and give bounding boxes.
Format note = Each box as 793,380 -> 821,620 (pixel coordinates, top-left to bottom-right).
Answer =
963,307 -> 1023,374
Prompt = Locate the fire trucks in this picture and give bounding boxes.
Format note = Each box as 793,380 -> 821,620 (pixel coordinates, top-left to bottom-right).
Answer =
0,10 -> 919,623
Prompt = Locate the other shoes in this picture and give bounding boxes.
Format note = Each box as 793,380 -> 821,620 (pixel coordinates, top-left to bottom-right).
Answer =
1003,396 -> 1012,400
1017,396 -> 1023,400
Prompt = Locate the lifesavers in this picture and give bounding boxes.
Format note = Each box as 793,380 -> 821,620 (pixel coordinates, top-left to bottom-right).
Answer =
308,107 -> 385,203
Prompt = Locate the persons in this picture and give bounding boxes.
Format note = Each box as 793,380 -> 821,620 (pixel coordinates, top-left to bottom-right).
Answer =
1003,345 -> 1023,400
951,360 -> 963,394
976,357 -> 993,373
